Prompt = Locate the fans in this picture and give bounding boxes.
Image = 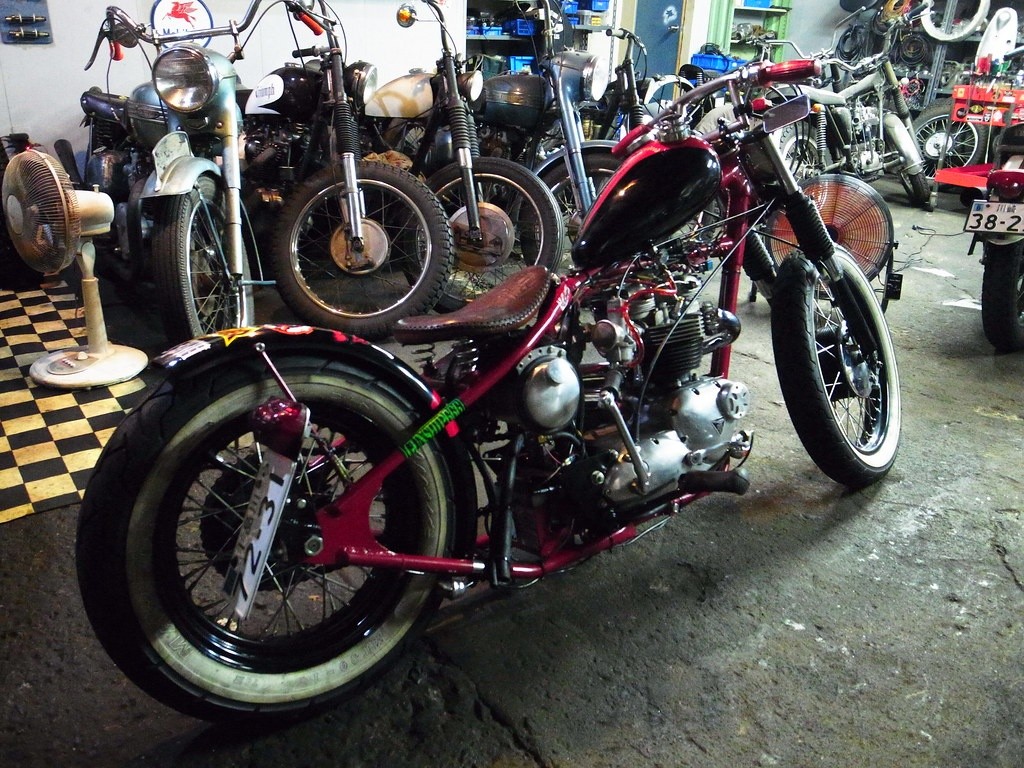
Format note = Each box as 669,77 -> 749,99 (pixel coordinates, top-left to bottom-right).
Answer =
750,173 -> 899,313
1,149 -> 148,391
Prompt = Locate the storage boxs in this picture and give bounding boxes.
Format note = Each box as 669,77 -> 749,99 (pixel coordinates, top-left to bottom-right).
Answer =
465,26 -> 503,36
688,54 -> 746,92
502,18 -> 536,36
467,54 -> 535,83
561,0 -> 609,26
743,0 -> 771,8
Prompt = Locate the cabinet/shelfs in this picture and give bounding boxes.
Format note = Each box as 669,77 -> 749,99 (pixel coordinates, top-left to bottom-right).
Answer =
466,0 -> 613,105
864,0 -> 1024,113
725,0 -> 793,97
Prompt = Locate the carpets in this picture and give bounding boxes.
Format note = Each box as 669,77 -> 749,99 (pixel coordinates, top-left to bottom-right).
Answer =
0,277 -> 147,522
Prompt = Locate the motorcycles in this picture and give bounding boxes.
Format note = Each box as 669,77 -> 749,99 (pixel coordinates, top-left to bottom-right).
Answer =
48,2 -> 1024,412
72,35 -> 901,741
